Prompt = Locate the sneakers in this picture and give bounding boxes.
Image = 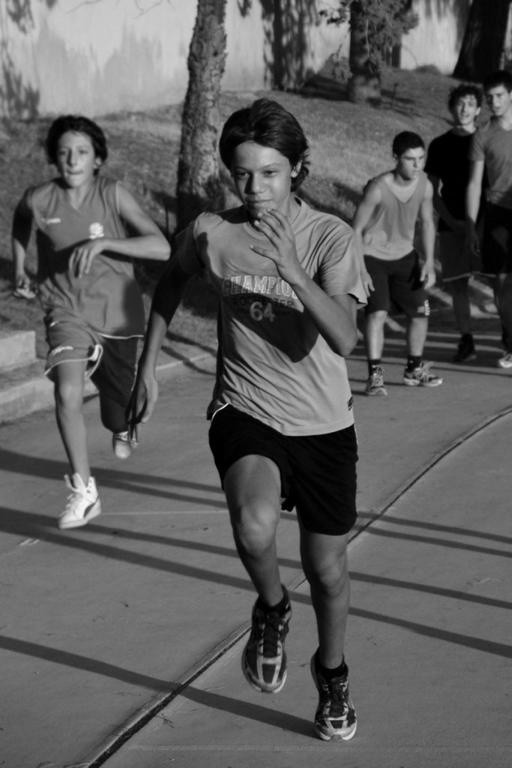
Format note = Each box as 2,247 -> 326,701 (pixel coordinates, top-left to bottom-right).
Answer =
450,345 -> 476,362
311,647 -> 358,742
242,584 -> 291,693
365,366 -> 387,396
497,353 -> 512,367
113,431 -> 137,458
403,368 -> 443,387
58,473 -> 101,530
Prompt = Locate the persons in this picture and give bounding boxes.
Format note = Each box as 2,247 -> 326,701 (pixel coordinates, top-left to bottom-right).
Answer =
11,115 -> 174,531
123,96 -> 379,743
352,127 -> 445,401
464,67 -> 511,372
420,82 -> 481,369
